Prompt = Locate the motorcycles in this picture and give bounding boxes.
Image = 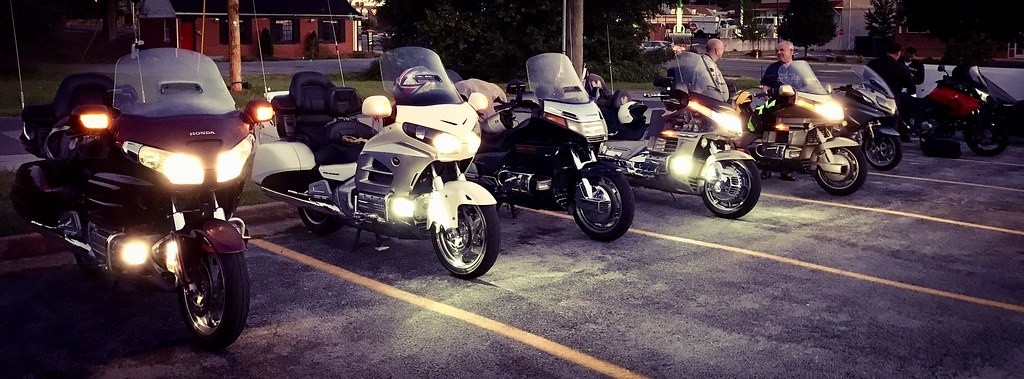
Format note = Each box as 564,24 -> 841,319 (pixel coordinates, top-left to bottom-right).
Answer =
898,60 -> 1009,158
827,62 -> 905,170
10,46 -> 278,351
722,58 -> 869,196
252,48 -> 502,280
583,50 -> 764,219
448,51 -> 635,242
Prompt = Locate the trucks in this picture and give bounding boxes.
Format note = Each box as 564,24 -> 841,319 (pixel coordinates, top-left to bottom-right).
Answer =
691,16 -> 739,37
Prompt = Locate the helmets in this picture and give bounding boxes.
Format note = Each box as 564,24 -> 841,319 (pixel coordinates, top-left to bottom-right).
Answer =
730,90 -> 754,117
391,66 -> 435,97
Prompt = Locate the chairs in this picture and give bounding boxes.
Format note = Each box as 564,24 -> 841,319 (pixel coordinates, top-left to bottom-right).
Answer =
21,72 -> 138,158
271,69 -> 363,152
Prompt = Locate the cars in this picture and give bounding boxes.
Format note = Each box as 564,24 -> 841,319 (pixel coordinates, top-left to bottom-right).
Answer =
641,40 -> 675,52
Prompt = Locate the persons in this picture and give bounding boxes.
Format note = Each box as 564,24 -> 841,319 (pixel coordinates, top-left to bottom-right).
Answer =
692,38 -> 729,102
864,43 -> 931,143
760,41 -> 808,179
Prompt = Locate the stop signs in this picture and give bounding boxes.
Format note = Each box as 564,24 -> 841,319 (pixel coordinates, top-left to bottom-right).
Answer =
838,28 -> 845,35
690,23 -> 697,31
